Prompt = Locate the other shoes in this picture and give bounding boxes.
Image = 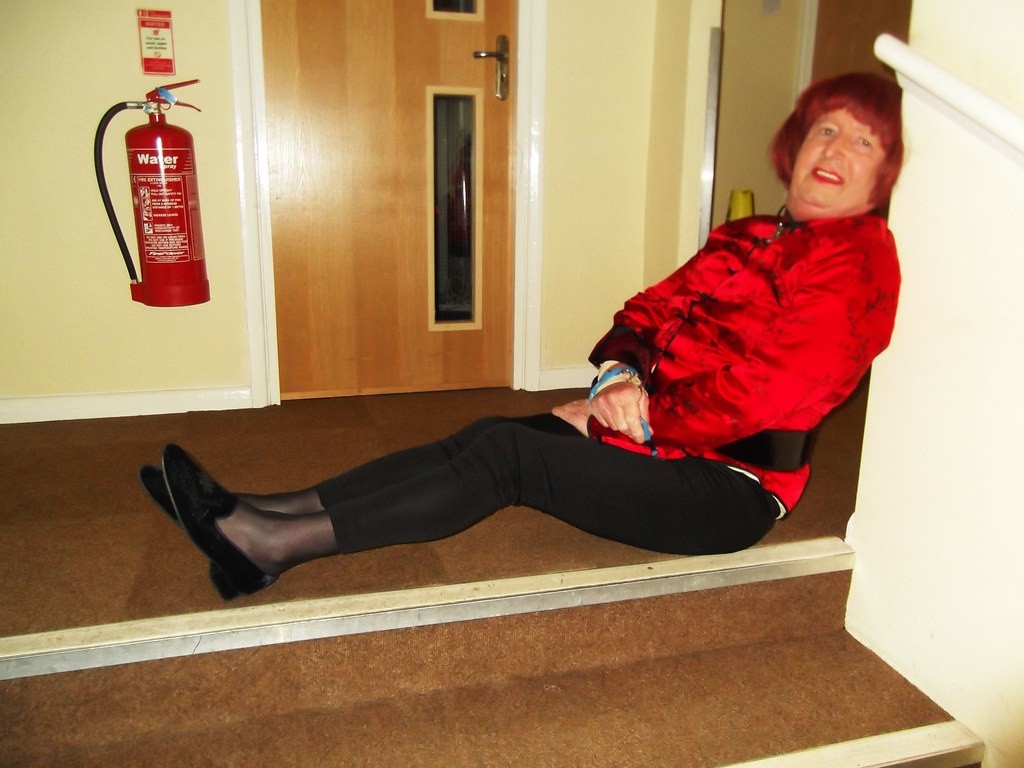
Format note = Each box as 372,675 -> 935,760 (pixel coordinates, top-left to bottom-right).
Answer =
139,442 -> 280,602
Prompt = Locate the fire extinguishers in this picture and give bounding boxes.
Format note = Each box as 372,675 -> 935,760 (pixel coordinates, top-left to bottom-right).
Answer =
92,78 -> 211,308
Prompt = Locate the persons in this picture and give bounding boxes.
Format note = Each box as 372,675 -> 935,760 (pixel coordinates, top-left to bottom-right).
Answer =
140,73 -> 902,600
